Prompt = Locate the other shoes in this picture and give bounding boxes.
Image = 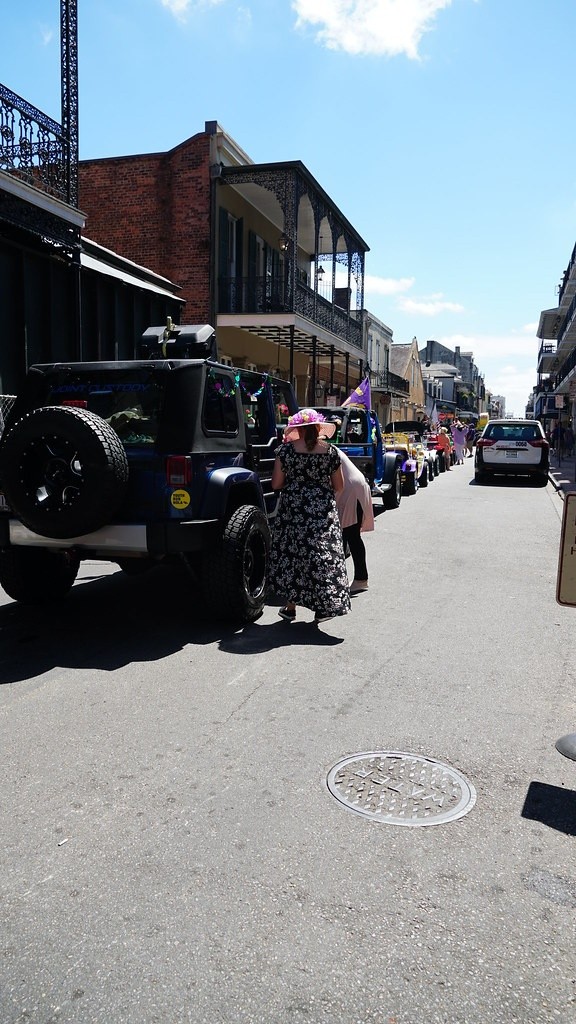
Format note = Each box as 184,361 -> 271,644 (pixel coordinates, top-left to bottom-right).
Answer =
447,469 -> 452,471
468,454 -> 473,458
456,463 -> 460,465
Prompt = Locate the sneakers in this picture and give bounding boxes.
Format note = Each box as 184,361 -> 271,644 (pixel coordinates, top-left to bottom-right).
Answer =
348,580 -> 369,592
315,612 -> 332,621
279,605 -> 296,620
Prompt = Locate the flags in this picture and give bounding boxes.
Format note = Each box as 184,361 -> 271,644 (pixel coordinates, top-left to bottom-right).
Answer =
343,378 -> 371,409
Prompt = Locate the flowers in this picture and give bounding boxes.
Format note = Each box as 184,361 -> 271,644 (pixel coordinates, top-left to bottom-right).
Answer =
287,412 -> 327,424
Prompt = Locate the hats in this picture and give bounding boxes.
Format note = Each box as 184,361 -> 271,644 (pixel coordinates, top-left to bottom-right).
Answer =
284,409 -> 336,439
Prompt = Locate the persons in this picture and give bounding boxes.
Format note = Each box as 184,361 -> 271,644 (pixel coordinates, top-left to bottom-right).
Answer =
421,415 -> 431,432
466,424 -> 477,457
546,422 -> 576,461
450,417 -> 469,465
334,445 -> 374,595
439,428 -> 452,471
264,409 -> 351,620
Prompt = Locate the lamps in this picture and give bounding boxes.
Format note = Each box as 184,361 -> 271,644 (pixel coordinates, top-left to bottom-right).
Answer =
278,232 -> 291,251
316,236 -> 325,281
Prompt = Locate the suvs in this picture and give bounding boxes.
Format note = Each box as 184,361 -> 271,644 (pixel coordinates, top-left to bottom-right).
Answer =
474,420 -> 550,484
0,359 -> 300,602
